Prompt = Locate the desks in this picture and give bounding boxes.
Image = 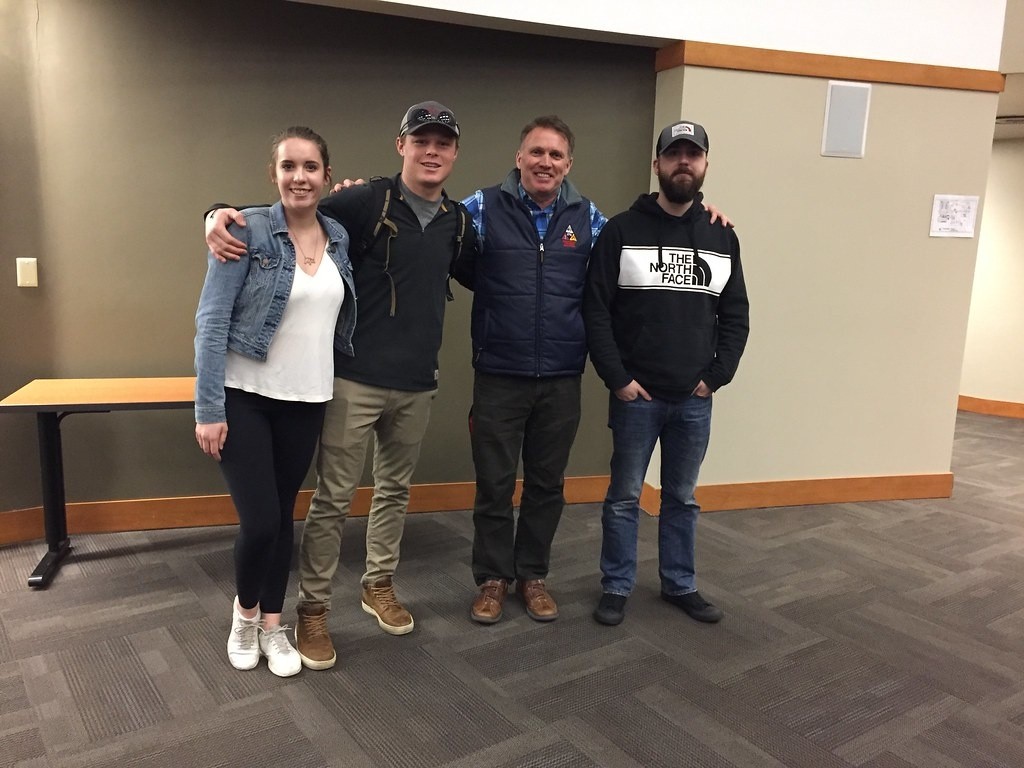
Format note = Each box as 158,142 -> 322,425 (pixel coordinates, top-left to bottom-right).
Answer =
1,376 -> 198,588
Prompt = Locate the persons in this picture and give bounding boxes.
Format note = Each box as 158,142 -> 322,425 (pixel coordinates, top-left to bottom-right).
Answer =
201,100 -> 480,671
191,126 -> 358,677
328,116 -> 735,624
580,121 -> 750,624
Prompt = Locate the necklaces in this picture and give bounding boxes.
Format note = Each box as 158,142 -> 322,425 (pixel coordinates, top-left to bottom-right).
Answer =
287,221 -> 319,266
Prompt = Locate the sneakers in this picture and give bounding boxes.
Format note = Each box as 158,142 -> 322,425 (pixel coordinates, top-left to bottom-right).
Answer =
258,628 -> 302,677
593,592 -> 627,626
226,595 -> 261,670
361,583 -> 415,636
294,607 -> 337,670
661,588 -> 722,622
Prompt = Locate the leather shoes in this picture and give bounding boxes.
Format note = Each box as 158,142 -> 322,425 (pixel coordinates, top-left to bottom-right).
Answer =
515,579 -> 559,620
470,578 -> 508,623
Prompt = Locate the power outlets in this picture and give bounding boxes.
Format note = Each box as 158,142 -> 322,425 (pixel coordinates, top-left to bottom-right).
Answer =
16,257 -> 39,288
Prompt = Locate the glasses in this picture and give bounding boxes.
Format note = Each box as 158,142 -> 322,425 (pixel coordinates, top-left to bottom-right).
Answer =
399,109 -> 460,137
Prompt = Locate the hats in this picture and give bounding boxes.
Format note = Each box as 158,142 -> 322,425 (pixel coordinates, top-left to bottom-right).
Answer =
656,121 -> 709,153
399,100 -> 461,140
